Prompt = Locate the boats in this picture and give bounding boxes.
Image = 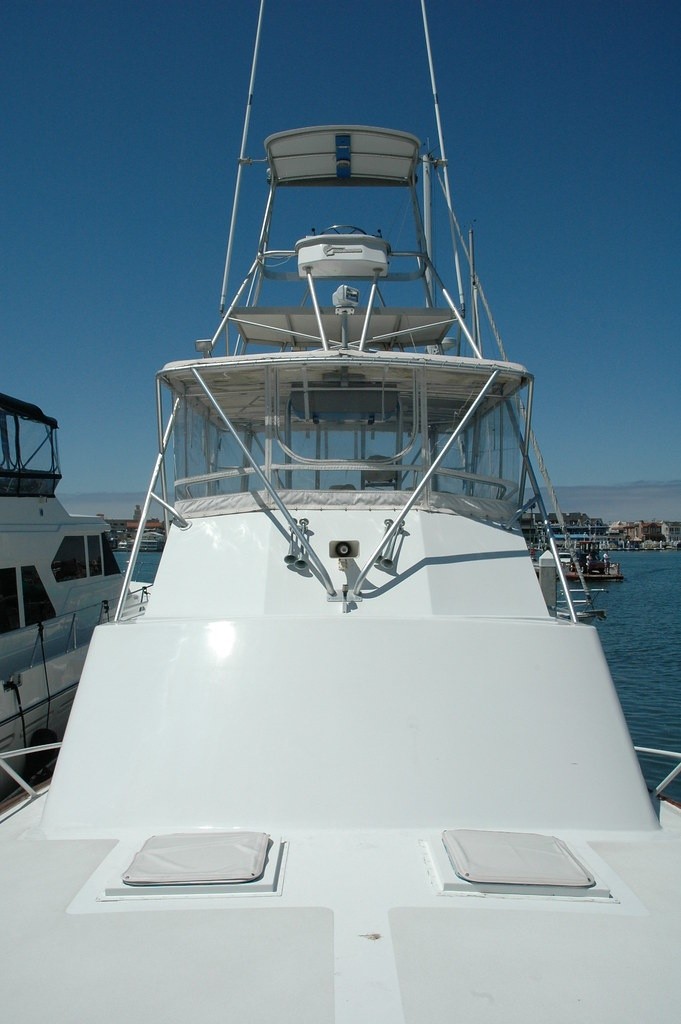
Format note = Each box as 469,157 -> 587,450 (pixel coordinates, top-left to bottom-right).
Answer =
0,391 -> 152,803
554,588 -> 610,626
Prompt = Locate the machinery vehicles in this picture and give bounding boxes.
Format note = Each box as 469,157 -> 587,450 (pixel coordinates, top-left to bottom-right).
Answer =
570,541 -> 605,575
